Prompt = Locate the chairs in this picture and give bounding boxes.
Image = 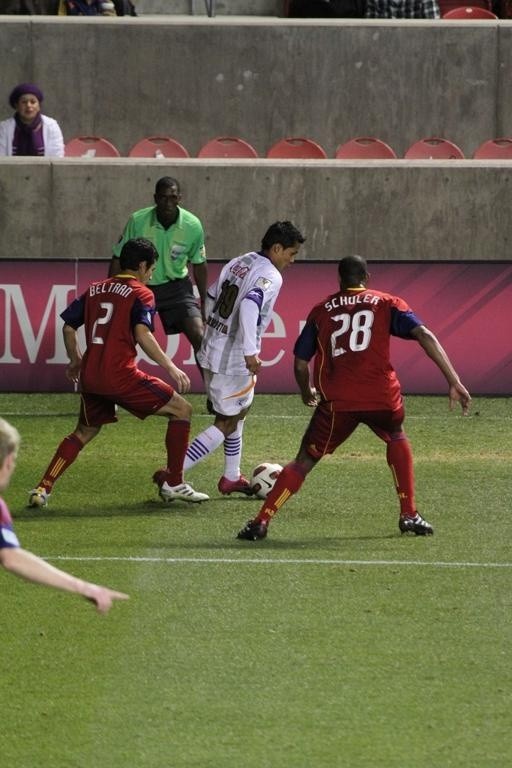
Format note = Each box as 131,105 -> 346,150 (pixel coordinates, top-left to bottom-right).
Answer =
128,135 -> 190,158
472,138 -> 512,159
442,7 -> 499,20
404,137 -> 464,160
197,136 -> 259,158
266,136 -> 328,159
65,136 -> 120,157
335,136 -> 397,160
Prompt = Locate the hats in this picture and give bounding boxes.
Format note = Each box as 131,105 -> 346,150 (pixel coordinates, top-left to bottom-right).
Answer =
8,83 -> 43,108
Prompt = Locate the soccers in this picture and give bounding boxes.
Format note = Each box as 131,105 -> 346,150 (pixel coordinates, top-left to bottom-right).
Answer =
249,462 -> 284,499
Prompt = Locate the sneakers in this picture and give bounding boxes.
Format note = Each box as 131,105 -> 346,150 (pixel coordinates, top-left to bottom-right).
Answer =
27,486 -> 52,508
158,480 -> 210,504
398,509 -> 435,535
152,470 -> 168,490
236,520 -> 268,541
217,474 -> 253,497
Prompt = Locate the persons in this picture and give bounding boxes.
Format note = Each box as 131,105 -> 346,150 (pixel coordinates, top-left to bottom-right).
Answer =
0,418 -> 130,615
363,0 -> 441,21
14,0 -> 137,21
29,236 -> 210,506
237,254 -> 472,541
0,82 -> 64,157
154,220 -> 306,496
108,176 -> 215,413
